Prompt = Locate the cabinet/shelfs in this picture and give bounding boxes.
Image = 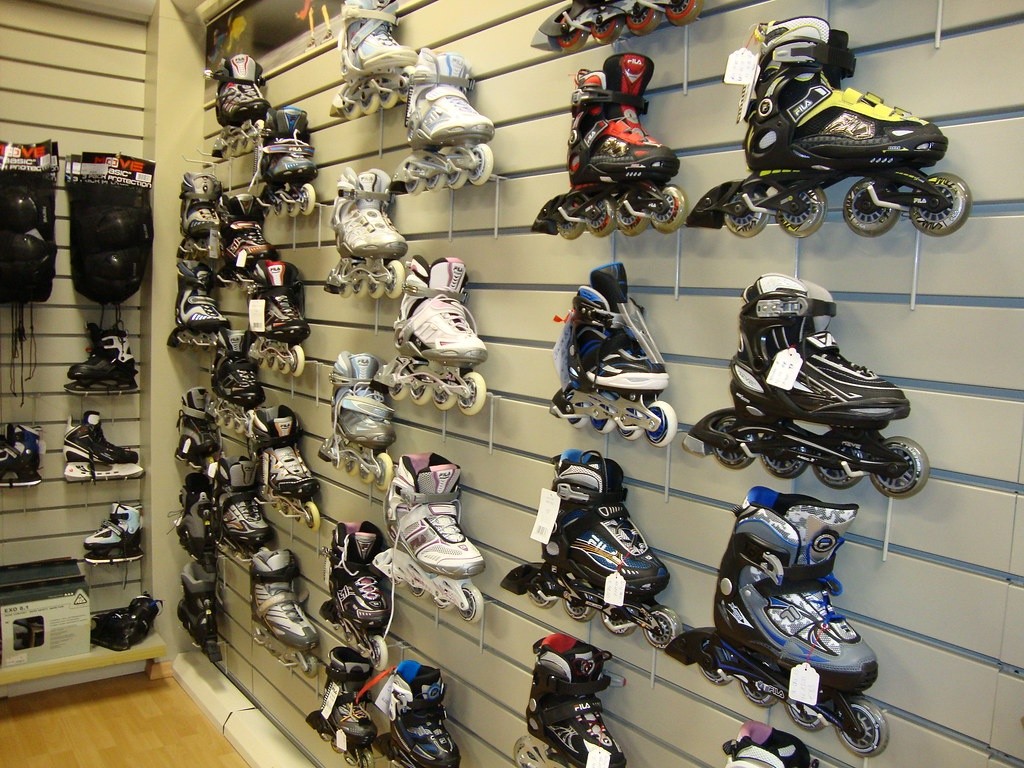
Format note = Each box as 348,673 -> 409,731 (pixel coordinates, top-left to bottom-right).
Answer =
166,0 -> 974,768
0,383 -> 146,563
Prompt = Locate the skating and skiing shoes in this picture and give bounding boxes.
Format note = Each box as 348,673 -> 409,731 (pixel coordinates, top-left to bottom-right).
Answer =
166,0 -> 495,768
499,0 -> 970,768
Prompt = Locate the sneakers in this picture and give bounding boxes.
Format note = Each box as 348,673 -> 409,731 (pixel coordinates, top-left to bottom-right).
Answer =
63,321 -> 142,397
82,501 -> 145,563
63,409 -> 146,482
0,423 -> 45,488
91,590 -> 159,653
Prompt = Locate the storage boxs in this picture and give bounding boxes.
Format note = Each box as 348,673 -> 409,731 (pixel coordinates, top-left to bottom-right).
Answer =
0,557 -> 91,667
